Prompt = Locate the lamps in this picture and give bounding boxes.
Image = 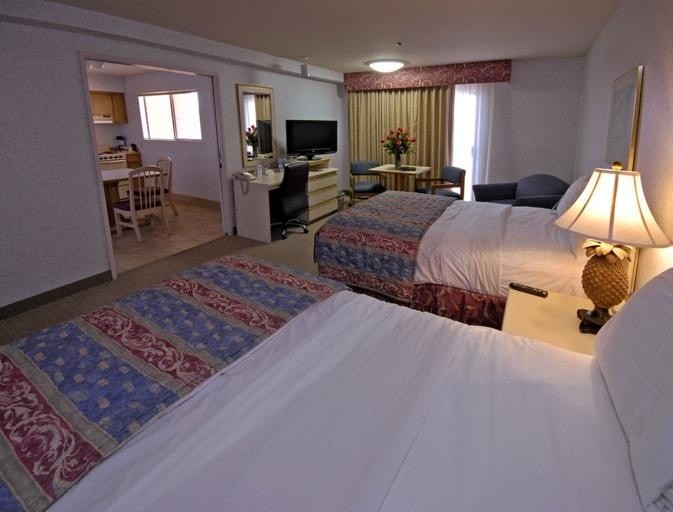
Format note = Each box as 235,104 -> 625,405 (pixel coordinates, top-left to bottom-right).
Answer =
94,64 -> 104,70
554,160 -> 673,328
365,59 -> 407,74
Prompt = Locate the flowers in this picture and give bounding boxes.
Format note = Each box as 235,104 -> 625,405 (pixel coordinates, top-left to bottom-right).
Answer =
380,127 -> 417,154
245,125 -> 258,146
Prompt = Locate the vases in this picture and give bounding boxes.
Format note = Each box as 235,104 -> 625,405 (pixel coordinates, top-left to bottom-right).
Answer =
394,153 -> 402,169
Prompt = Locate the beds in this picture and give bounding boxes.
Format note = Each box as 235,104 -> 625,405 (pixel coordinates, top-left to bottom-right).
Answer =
314,190 -> 587,330
0,255 -> 673,512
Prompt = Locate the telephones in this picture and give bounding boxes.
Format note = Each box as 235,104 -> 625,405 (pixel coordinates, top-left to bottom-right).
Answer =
234,172 -> 256,181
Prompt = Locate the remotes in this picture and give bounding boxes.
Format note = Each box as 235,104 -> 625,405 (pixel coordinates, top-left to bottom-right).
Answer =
509,281 -> 548,298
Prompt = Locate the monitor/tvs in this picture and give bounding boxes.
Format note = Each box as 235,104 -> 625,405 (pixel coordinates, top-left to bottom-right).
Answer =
255,120 -> 273,157
285,120 -> 338,161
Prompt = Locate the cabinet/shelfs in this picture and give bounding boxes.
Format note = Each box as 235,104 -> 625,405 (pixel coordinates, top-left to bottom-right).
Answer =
112,92 -> 127,123
126,153 -> 144,189
90,91 -> 113,116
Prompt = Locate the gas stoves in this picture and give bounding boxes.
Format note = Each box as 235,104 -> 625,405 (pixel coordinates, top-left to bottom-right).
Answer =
98,151 -> 124,155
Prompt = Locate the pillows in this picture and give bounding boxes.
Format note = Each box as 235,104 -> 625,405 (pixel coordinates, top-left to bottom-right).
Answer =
595,268 -> 673,509
569,232 -> 587,257
556,174 -> 590,217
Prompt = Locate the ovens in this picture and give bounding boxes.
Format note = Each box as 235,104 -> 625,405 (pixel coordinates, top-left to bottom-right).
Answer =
99,159 -> 127,171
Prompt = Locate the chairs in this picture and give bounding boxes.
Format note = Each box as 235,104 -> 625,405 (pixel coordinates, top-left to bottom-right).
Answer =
473,174 -> 570,209
113,166 -> 172,242
351,160 -> 388,205
414,166 -> 466,200
271,162 -> 310,239
127,155 -> 178,216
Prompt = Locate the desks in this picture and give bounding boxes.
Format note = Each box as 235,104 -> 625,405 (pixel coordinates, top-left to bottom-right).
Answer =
233,158 -> 339,243
368,164 -> 432,192
102,168 -> 167,234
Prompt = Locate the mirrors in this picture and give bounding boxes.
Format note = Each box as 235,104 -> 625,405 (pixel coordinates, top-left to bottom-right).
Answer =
237,82 -> 277,168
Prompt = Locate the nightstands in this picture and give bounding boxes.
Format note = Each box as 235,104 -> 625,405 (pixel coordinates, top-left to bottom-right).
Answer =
501,288 -> 613,356
